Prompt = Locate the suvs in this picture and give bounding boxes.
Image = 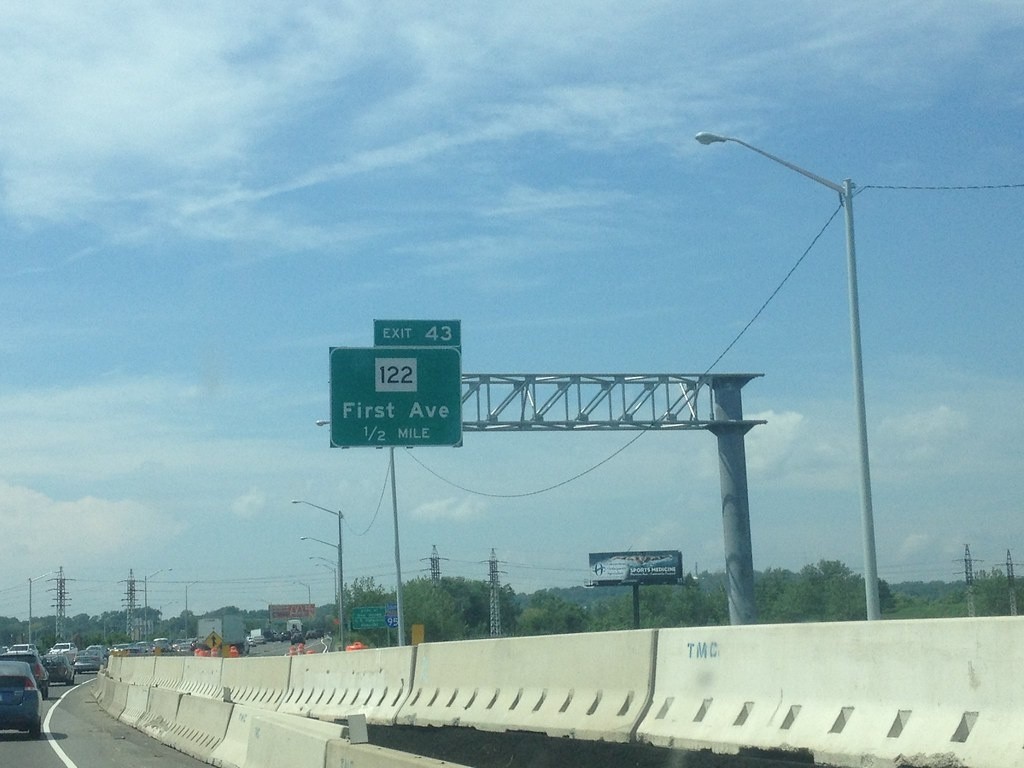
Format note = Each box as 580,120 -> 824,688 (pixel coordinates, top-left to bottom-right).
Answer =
0,650 -> 51,700
50,643 -> 79,657
0,644 -> 41,657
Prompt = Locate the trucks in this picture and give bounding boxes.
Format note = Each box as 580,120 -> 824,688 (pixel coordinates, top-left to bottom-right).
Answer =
196,614 -> 245,653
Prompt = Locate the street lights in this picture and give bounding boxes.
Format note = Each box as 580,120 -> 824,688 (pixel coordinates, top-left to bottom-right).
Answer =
293,580 -> 311,631
185,580 -> 199,639
292,499 -> 345,653
693,131 -> 881,621
316,420 -> 404,647
29,570 -> 62,643
145,568 -> 173,642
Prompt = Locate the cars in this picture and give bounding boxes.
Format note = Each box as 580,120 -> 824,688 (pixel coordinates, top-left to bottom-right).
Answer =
74,650 -> 108,674
247,628 -> 325,647
0,660 -> 43,740
43,654 -> 76,686
87,637 -> 197,659
